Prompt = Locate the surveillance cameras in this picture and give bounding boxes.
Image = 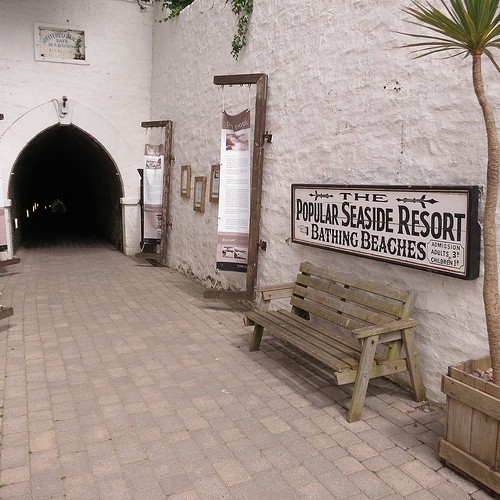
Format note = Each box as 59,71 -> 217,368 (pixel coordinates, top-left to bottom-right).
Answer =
61,96 -> 68,114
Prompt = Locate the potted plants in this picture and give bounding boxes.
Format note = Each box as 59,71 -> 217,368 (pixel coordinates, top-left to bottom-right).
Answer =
386,1 -> 499,498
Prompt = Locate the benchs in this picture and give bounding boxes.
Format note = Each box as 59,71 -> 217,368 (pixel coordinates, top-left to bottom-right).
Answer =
243,260 -> 430,423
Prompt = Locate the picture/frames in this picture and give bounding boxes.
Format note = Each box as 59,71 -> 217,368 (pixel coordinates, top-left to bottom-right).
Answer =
33,22 -> 90,65
